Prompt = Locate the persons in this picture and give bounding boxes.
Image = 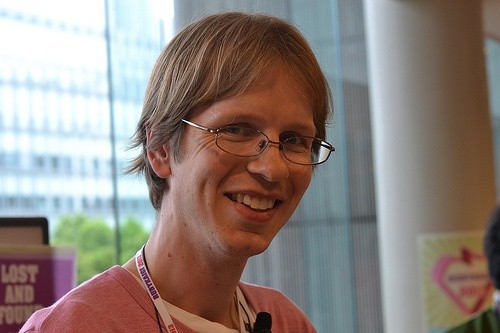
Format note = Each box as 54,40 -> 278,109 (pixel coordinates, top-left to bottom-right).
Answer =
448,205 -> 500,333
19,13 -> 336,333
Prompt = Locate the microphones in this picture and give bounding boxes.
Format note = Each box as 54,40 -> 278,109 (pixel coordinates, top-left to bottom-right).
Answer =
253,312 -> 272,333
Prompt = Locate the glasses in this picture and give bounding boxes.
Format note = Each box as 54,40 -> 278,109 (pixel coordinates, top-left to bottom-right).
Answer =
177,119 -> 335,166
